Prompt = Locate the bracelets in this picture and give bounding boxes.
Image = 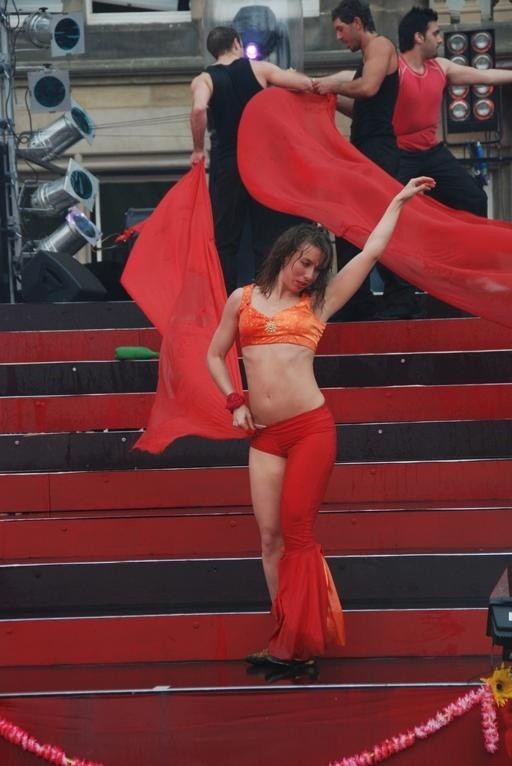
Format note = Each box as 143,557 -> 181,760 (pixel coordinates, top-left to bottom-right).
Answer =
225,392 -> 247,414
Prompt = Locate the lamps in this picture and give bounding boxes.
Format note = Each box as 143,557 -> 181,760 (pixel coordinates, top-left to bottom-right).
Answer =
241,29 -> 274,60
486,595 -> 512,660
0,1 -> 100,305
444,21 -> 497,135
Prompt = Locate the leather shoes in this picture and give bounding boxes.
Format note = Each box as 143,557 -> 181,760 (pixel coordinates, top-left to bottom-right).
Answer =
245,653 -> 317,668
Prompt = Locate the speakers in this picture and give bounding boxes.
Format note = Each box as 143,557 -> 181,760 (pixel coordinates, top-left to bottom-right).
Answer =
22,250 -> 108,302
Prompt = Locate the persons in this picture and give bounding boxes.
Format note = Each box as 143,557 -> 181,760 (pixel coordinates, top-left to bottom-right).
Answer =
190,26 -> 314,280
391,6 -> 512,219
313,0 -> 400,181
206,176 -> 437,681
